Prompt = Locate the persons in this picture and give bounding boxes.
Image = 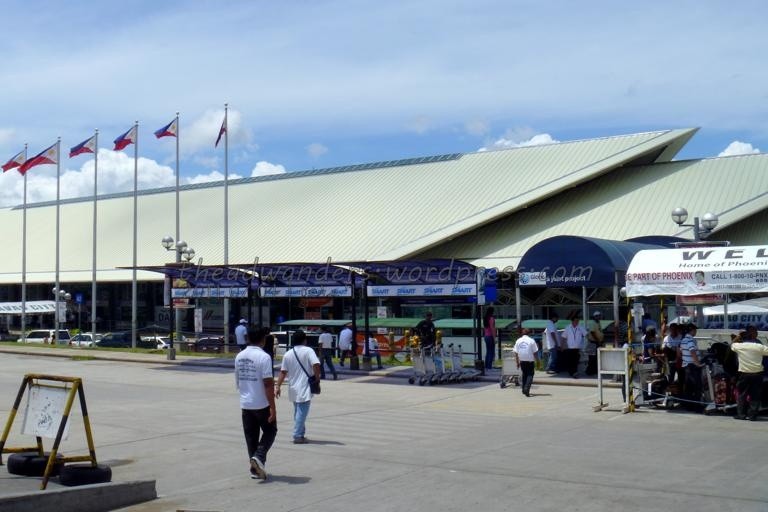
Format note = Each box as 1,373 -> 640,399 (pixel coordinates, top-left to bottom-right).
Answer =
512,328 -> 539,397
259,327 -> 274,378
337,322 -> 357,366
234,319 -> 250,352
317,324 -> 337,380
695,271 -> 706,289
586,311 -> 768,421
484,307 -> 497,370
274,329 -> 321,444
543,313 -> 561,375
234,326 -> 277,481
363,334 -> 383,369
415,311 -> 436,358
560,317 -> 587,378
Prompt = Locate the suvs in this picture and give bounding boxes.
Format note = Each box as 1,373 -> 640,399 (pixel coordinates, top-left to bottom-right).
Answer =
17,329 -> 71,346
70,334 -> 144,348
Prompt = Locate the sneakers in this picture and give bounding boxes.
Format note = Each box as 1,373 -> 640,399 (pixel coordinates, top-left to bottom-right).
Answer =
735,415 -> 756,420
250,449 -> 266,479
523,388 -> 529,396
293,435 -> 308,443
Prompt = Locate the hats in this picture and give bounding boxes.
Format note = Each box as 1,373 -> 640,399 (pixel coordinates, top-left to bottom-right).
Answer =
240,319 -> 248,323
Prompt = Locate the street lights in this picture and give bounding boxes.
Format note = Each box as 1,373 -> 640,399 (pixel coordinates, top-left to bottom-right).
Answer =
52,287 -> 72,329
669,206 -> 718,328
163,235 -> 194,343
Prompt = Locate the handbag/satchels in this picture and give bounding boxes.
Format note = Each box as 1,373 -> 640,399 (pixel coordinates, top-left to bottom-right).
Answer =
309,376 -> 320,393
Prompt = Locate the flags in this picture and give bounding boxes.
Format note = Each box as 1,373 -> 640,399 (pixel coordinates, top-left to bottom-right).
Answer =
1,148 -> 26,173
214,115 -> 225,148
69,136 -> 96,159
113,127 -> 136,153
16,142 -> 58,176
153,117 -> 177,140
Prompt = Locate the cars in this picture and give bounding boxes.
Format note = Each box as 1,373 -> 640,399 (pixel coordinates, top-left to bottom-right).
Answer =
188,335 -> 241,353
143,337 -> 176,350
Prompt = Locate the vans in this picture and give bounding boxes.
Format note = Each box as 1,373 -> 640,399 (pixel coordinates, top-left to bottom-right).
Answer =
268,331 -> 321,359
